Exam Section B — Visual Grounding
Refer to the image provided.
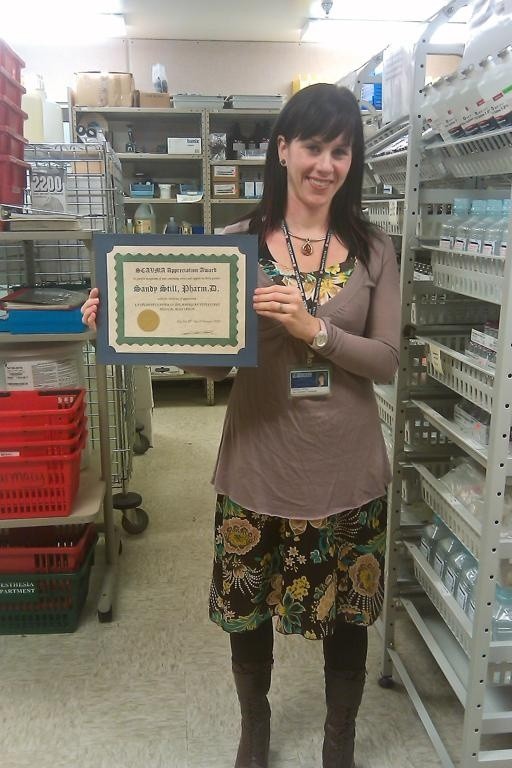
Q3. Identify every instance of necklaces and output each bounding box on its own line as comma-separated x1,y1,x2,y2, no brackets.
281,218,338,259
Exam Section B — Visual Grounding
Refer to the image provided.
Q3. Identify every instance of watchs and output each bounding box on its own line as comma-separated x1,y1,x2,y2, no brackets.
307,317,328,351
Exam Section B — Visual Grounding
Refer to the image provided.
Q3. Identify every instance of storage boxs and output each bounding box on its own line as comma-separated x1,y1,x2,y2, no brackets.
1,39,31,204
74,70,133,107
212,165,240,181
138,91,170,108
212,182,240,199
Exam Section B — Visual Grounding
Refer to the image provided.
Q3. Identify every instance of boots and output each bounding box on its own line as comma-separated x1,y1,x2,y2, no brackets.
322,666,365,768
233,665,271,767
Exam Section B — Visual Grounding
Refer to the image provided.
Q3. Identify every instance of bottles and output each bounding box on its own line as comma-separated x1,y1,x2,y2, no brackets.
420,516,512,684
420,45,512,150
438,194,512,254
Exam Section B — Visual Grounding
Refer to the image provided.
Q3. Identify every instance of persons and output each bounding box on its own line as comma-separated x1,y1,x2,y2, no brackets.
81,81,404,768
316,373,326,387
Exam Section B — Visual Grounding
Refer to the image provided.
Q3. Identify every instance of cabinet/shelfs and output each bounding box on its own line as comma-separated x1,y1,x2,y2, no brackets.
64,103,293,404
0,226,125,626
336,122,512,766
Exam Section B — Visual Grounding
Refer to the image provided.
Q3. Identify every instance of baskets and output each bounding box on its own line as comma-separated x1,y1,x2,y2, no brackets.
0,387,86,519
0,37,29,213
0,532,100,633
1,521,96,609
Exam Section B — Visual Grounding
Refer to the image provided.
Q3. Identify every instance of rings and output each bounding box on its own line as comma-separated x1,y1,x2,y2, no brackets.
278,301,285,314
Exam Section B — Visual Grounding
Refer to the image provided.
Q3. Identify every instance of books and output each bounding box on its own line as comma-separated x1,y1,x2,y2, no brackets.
0,285,90,313
